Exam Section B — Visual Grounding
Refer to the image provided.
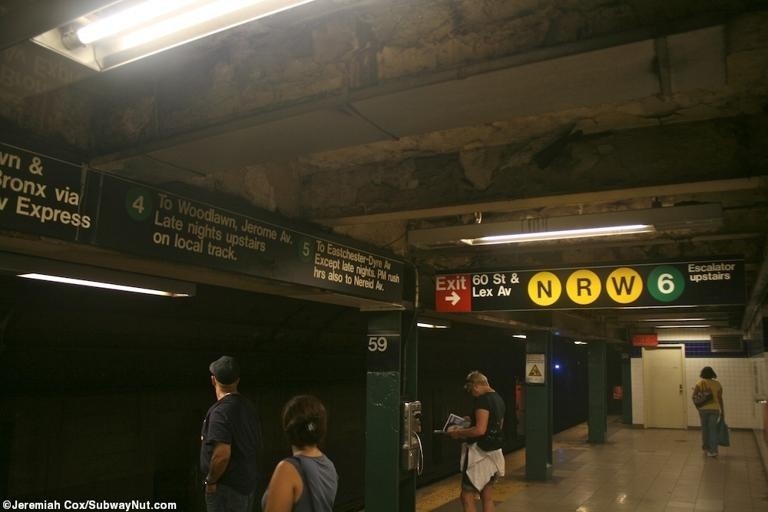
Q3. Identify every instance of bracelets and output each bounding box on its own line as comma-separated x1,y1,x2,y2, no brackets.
203,477,217,486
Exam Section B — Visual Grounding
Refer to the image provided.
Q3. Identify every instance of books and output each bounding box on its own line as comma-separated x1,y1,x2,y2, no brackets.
434,412,471,434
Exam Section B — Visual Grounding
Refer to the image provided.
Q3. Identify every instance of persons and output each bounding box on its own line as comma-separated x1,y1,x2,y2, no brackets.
447,370,506,511
445,423,461,432
692,366,724,458
260,396,340,511
198,355,260,511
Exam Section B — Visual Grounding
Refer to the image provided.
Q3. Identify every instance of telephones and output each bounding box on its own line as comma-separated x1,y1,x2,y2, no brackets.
401,400,422,471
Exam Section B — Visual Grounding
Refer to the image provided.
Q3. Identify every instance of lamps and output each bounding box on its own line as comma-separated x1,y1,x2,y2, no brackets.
407,204,724,249
0,251,197,297
30,0,320,72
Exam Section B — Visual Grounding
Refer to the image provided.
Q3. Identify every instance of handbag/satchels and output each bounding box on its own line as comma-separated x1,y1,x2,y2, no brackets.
693,380,713,406
717,417,729,446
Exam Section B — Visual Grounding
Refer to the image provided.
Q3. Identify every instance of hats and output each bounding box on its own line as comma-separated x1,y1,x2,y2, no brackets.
209,355,241,384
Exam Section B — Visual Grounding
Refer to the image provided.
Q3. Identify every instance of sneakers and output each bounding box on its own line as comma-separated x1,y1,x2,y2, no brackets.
707,451,718,457
